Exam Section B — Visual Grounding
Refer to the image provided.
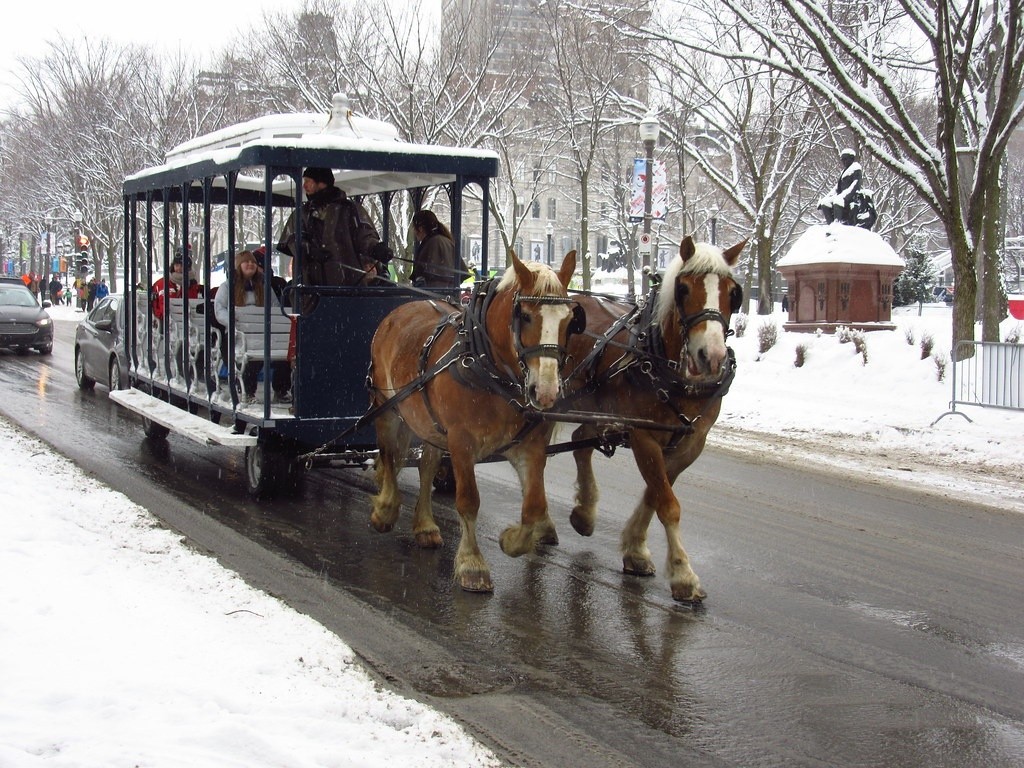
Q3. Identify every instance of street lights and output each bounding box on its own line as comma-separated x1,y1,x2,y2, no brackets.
638,109,662,293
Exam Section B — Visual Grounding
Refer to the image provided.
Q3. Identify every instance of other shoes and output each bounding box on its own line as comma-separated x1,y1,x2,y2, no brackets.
274,391,294,403
244,392,262,404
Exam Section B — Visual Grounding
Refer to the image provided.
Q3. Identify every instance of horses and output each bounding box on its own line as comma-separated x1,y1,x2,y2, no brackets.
370,235,753,604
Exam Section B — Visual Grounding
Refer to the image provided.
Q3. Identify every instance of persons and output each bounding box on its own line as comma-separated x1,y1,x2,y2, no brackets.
817,148,863,225
22,271,109,312
782,294,789,312
276,166,473,288
149,239,293,405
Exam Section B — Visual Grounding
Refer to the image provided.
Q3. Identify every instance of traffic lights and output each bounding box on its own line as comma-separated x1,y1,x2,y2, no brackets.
79,235,88,273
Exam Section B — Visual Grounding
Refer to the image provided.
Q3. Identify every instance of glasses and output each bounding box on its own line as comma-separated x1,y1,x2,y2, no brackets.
174,252,192,259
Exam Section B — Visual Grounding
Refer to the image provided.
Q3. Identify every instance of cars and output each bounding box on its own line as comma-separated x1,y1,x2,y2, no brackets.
73,291,129,401
0,277,54,355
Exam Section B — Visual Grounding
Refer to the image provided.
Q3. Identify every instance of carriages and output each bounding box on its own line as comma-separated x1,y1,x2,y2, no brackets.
107,92,753,605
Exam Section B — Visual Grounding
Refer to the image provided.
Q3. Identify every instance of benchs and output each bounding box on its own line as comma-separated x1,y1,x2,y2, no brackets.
135,292,292,411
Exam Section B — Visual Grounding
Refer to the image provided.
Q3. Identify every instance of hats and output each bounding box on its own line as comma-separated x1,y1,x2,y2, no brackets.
252,247,266,265
303,166,334,187
236,249,258,268
